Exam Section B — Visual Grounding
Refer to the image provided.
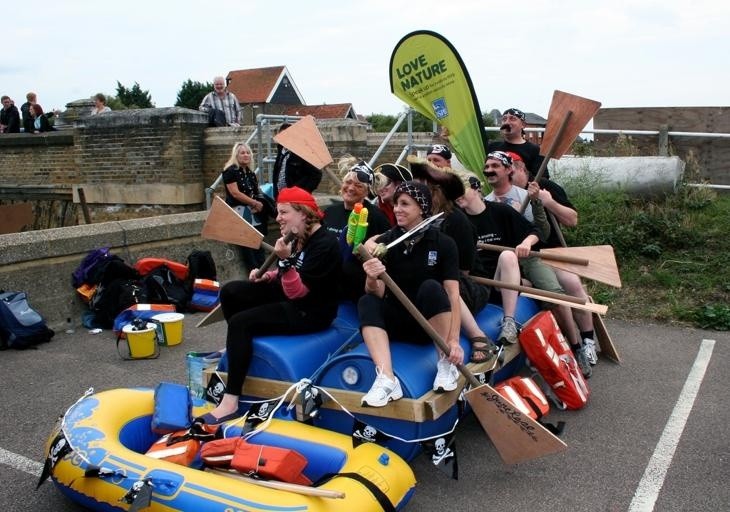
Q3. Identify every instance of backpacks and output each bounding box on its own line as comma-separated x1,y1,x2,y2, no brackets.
183,247,221,313
0,287,55,352
145,263,190,315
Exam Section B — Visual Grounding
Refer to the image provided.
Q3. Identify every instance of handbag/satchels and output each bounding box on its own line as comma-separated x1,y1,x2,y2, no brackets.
71,248,141,330
208,108,232,128
112,303,180,347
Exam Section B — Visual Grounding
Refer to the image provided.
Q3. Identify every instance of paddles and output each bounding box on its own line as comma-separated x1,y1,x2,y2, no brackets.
356,243,568,466
476,241,622,288
518,90,601,215
460,271,609,315
201,195,274,252
195,226,298,327
272,115,343,190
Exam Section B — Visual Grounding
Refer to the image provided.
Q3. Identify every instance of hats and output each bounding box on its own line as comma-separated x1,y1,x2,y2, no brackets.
371,162,415,184
277,183,315,203
406,154,466,202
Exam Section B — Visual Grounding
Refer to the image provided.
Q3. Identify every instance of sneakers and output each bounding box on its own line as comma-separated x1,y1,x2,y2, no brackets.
573,347,594,379
496,315,518,345
360,363,404,407
431,349,461,393
195,407,243,425
583,337,599,365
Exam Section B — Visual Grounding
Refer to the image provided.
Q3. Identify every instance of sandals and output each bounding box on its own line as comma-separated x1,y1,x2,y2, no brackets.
468,335,498,363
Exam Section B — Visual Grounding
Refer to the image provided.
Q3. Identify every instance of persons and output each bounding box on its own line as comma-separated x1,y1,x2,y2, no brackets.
323,144,542,408
21,93,37,132
195,186,343,425
224,124,322,273
90,93,111,116
483,108,598,378
28,103,52,134
199,75,242,127
0,95,20,133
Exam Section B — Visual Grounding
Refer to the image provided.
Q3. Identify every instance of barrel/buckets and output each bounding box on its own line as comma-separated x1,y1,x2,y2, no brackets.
152,312,185,346
120,321,159,358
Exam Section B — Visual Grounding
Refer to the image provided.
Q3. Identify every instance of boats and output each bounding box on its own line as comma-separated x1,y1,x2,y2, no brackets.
41,386,419,510
200,286,547,463
